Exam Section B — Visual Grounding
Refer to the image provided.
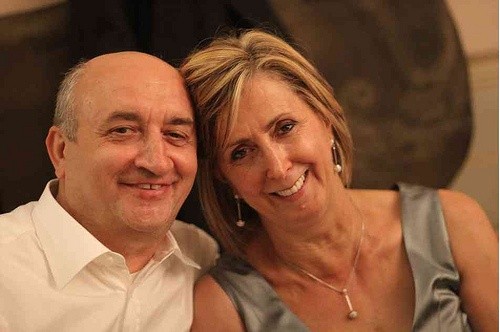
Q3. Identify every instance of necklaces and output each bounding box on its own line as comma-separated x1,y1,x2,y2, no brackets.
282,190,365,319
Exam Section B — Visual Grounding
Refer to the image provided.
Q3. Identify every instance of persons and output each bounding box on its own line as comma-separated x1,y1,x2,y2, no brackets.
0,51,220,332
178,22,500,332
